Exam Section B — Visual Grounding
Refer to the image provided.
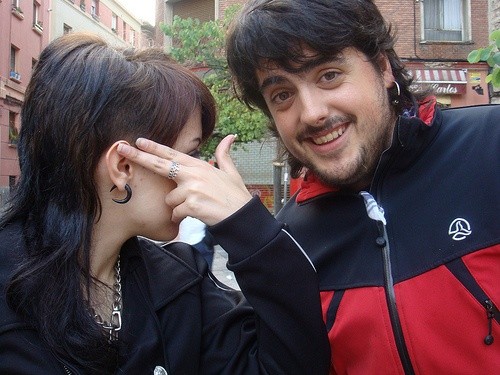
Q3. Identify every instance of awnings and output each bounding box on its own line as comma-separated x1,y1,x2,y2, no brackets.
404,68,467,95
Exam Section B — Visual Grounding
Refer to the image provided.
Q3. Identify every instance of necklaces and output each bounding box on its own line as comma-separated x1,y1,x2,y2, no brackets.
76,258,125,342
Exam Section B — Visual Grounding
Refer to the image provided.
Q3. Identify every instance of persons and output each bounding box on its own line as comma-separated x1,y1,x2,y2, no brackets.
0,34,334,375
226,0,500,375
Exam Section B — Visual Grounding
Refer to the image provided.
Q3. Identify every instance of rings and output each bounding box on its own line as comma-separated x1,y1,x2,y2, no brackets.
169,161,181,181
